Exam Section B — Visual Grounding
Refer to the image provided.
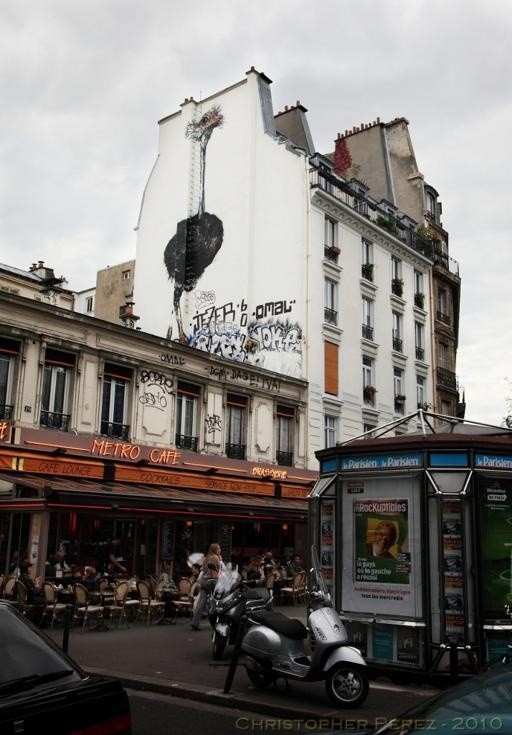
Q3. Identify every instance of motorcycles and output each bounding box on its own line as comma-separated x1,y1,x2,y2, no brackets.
240,587,370,710
203,563,277,660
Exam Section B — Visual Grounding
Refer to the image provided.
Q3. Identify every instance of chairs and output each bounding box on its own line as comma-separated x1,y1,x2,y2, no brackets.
0,557,309,635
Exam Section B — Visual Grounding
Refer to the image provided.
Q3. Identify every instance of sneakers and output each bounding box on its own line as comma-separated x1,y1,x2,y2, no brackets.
191,624,199,631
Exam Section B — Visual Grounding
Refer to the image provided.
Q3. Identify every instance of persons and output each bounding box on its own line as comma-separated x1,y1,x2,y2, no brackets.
192,541,224,634
368,522,399,560
1,550,309,625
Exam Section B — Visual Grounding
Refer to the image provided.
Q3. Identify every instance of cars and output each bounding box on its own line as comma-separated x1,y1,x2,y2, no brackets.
378,657,511,734
1,602,135,735
